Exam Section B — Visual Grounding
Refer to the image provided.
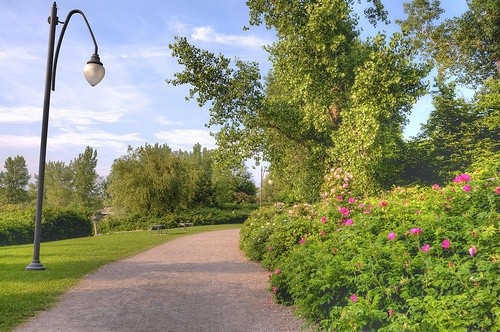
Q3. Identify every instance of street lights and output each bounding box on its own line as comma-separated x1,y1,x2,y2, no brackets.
24,2,106,275
259,164,270,207
